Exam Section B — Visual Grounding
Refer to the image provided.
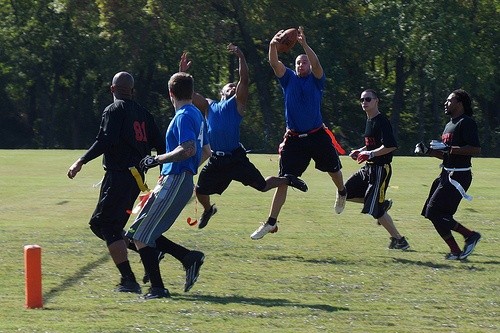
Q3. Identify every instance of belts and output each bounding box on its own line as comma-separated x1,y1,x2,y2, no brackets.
289,126,324,137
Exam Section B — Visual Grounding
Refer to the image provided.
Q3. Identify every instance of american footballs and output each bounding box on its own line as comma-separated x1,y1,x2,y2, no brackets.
278,28,299,53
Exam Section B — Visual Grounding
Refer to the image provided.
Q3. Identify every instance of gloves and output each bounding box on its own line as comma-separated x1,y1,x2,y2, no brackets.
414,143,430,154
430,140,453,154
357,151,373,164
140,155,161,174
349,149,362,160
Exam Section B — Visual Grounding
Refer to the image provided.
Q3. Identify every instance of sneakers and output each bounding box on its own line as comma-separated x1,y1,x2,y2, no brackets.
250,221,278,239
387,236,410,251
444,251,466,261
284,174,308,192
378,199,392,225
114,276,142,295
463,231,481,256
335,189,347,214
138,287,170,300
199,203,217,229
139,251,166,284
182,250,205,292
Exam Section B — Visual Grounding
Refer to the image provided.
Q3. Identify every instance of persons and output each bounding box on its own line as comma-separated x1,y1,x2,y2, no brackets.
341,89,410,251
67,72,166,292
422,89,482,261
177,43,309,229
248,27,346,241
124,72,205,299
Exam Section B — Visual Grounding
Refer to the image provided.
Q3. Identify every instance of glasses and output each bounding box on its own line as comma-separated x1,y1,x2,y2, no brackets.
359,97,376,102
